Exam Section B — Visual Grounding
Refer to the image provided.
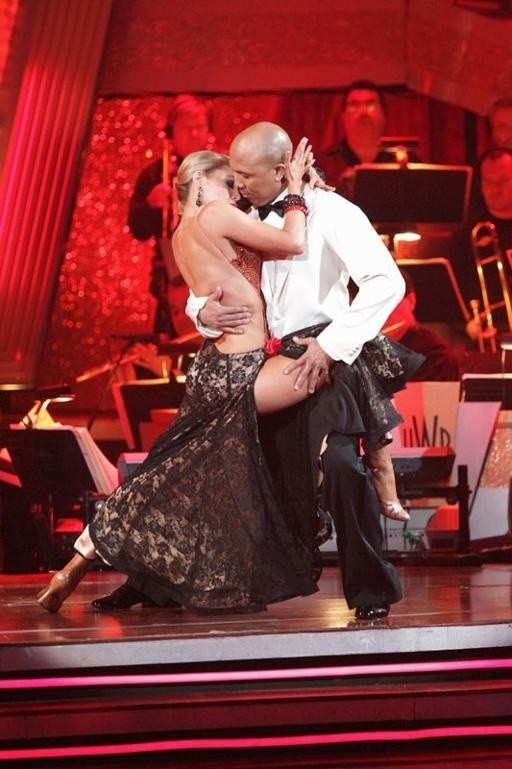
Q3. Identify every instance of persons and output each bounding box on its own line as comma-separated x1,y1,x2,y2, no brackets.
35,135,413,615
91,120,410,619
483,94,511,154
125,94,213,337
308,76,424,190
441,142,512,308
125,271,209,378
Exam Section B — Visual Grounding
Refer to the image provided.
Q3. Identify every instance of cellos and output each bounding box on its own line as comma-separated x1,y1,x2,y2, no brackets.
155,123,208,352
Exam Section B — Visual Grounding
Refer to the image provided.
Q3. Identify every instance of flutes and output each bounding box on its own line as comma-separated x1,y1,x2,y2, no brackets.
75,346,154,383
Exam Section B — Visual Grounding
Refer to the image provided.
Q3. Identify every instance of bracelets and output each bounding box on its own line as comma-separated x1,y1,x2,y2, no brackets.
282,194,309,217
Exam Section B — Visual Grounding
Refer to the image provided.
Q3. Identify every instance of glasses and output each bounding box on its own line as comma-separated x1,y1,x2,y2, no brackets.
343,97,379,113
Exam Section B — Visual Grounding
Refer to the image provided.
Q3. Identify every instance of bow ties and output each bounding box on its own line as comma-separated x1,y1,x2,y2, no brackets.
256,200,288,220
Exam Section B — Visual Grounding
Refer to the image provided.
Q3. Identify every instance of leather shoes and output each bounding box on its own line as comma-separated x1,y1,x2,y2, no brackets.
92,583,152,610
366,458,410,523
354,601,390,620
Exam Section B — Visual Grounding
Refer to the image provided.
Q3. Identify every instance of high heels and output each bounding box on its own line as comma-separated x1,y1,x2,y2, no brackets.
36,568,74,613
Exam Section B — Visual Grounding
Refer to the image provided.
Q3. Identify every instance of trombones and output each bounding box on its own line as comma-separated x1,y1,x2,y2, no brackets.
471,221,512,352
